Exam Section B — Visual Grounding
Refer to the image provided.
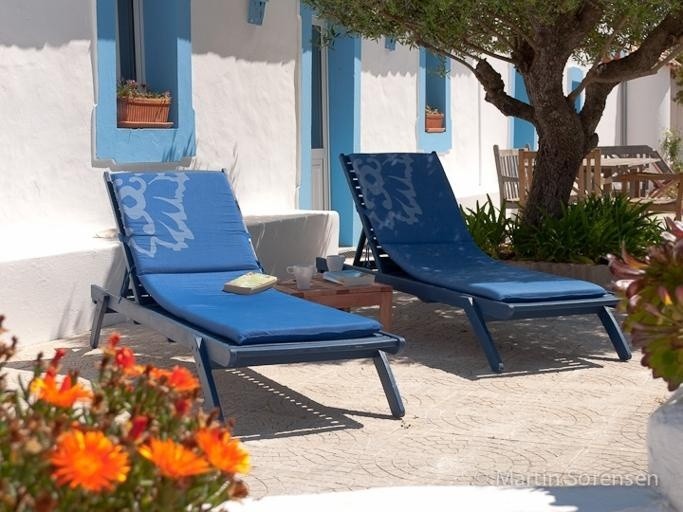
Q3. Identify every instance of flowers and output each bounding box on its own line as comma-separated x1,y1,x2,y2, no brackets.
1,314,253,511
116,77,170,99
425,104,441,114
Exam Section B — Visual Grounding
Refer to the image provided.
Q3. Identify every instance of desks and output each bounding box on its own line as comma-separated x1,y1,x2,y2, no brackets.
269,275,392,333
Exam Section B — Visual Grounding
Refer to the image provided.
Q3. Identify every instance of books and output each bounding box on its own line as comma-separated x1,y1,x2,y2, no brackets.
322,268,375,287
223,272,277,295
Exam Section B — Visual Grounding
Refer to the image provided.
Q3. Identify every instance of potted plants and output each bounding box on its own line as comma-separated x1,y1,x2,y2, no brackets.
607,216,683,512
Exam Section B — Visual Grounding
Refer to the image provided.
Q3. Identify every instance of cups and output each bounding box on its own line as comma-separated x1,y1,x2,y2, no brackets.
326,255,346,273
287,264,314,290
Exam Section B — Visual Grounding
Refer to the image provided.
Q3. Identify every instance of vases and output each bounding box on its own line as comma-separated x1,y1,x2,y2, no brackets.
425,113,446,133
117,96,175,128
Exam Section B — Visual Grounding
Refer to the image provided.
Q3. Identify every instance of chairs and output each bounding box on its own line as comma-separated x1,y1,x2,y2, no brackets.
340,150,634,372
87,168,405,433
492,143,681,248
